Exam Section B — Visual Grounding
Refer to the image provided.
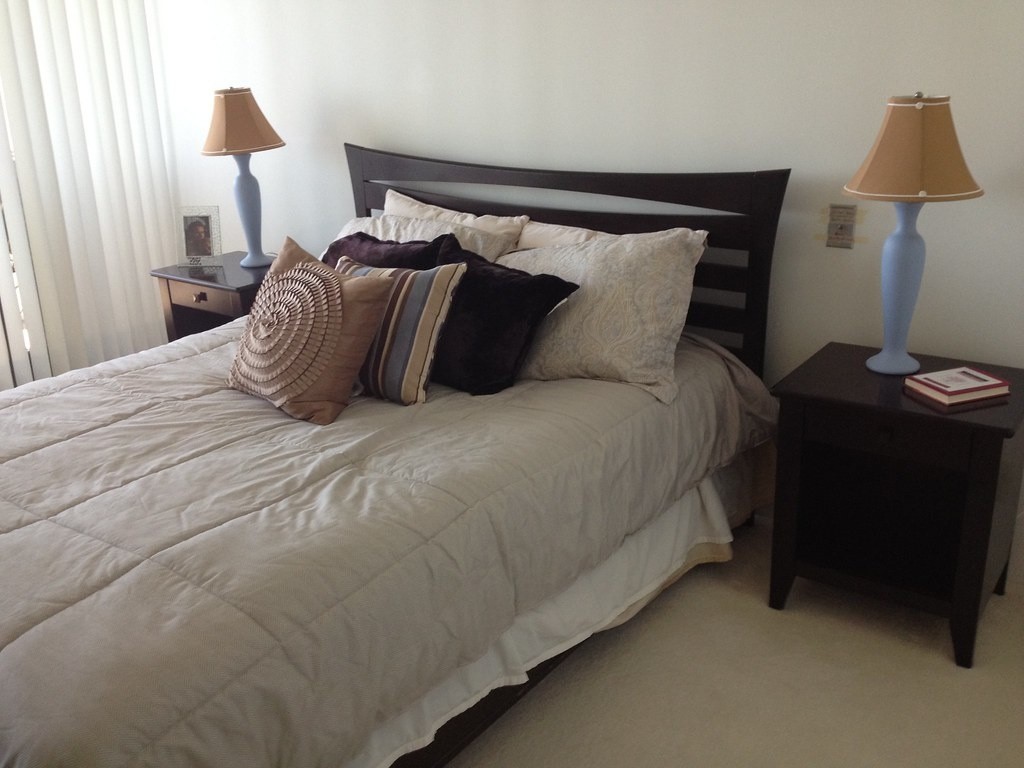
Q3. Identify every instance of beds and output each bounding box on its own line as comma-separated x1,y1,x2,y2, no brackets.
0,143,793,768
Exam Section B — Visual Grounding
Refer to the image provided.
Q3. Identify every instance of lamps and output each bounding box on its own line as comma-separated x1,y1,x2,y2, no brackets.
841,91,984,375
201,87,287,268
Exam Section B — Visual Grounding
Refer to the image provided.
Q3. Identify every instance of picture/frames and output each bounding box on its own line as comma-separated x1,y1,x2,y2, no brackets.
174,205,223,268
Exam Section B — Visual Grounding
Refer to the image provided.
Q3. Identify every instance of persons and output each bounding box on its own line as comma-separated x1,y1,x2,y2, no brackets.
185,218,211,256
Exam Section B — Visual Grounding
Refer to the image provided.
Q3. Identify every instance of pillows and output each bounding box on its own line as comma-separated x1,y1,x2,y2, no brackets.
225,235,396,425
495,227,710,406
435,233,580,397
318,189,530,263
334,255,468,407
321,230,448,271
518,220,613,250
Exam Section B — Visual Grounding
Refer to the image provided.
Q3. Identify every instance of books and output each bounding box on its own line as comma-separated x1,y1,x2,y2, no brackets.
902,366,1011,407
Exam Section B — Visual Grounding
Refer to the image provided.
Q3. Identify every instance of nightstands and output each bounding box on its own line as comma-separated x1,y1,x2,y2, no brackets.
769,341,1024,669
150,251,273,343
